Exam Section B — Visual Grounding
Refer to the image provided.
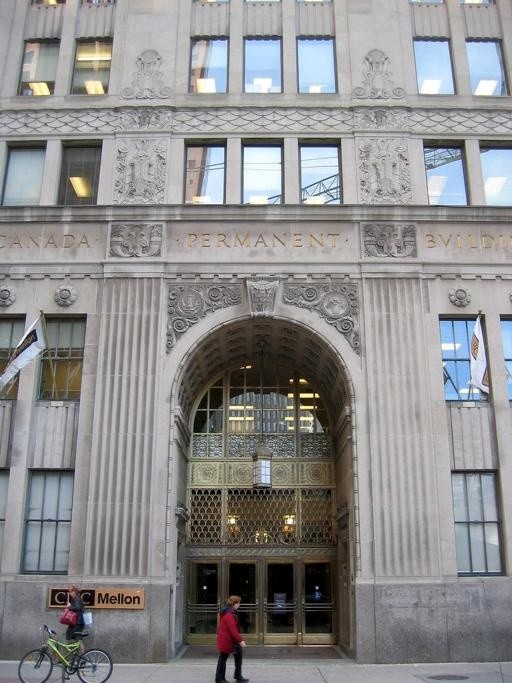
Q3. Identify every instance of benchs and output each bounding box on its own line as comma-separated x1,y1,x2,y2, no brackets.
0,314,46,390
470,316,490,394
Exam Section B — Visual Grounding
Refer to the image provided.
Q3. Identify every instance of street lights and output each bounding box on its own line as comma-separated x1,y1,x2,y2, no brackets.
83,612,93,626
58,608,79,626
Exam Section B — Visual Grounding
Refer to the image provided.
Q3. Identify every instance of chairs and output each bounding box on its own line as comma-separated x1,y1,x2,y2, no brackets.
216,679,229,683
234,674,248,682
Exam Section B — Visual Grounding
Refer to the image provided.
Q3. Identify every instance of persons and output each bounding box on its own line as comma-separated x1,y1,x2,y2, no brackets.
215,596,249,683
66,586,84,656
